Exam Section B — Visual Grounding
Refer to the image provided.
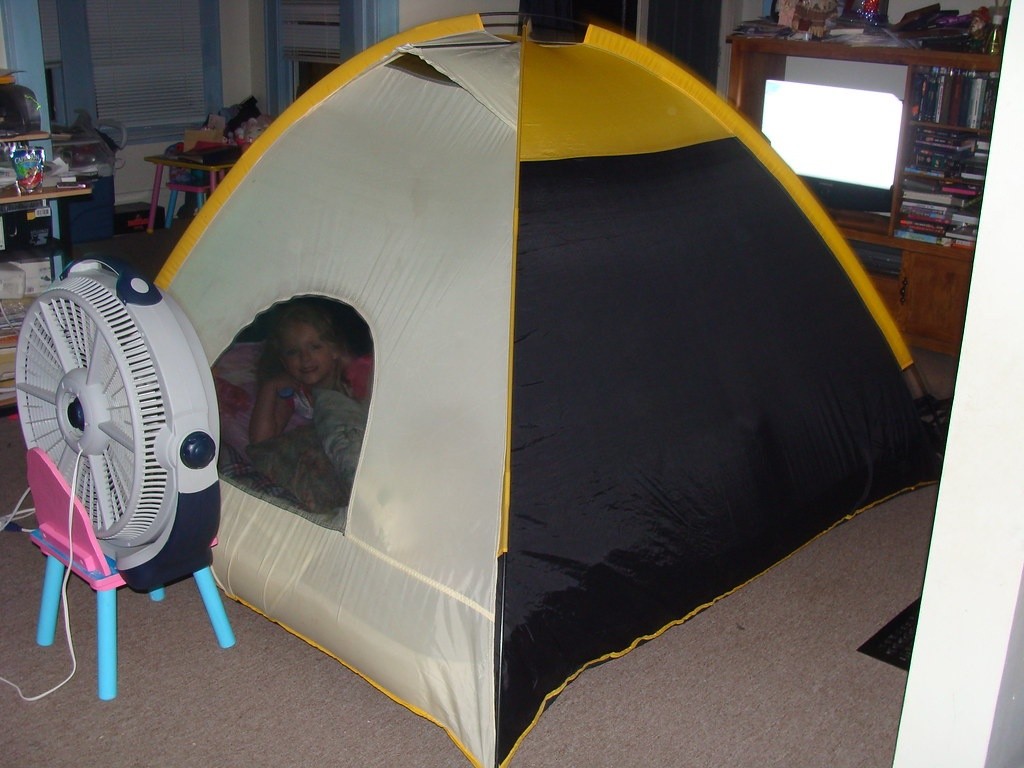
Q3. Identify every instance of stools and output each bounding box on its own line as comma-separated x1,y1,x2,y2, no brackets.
166,182,209,229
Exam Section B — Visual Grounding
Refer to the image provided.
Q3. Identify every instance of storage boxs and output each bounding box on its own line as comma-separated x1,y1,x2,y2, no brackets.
57,176,166,245
8,256,52,295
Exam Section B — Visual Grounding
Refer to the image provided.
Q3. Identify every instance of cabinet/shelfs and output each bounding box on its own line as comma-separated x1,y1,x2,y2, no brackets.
0,132,93,203
725,34,1003,359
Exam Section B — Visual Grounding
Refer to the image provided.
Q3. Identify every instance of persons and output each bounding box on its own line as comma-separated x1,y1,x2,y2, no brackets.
246,300,375,445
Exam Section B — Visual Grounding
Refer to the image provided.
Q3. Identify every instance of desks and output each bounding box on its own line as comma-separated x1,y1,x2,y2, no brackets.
144,155,237,233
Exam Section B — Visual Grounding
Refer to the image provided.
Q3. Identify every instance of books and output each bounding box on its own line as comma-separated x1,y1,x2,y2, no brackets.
822,10,903,48
0,295,48,404
894,64,999,249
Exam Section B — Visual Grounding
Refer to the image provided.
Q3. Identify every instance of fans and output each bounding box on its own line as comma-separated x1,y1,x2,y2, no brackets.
15,250,222,593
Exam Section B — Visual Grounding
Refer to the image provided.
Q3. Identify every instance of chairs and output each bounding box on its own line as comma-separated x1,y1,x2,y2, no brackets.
25,447,237,701
